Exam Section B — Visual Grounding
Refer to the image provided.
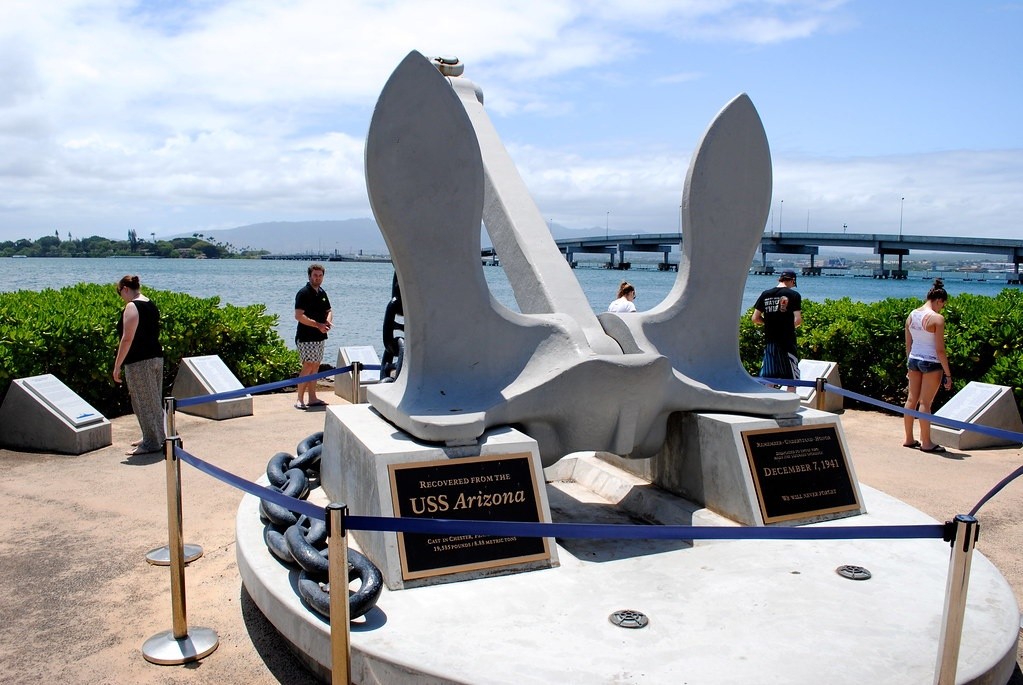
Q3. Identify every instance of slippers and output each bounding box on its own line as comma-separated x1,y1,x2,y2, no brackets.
295,402,309,409
903,440,921,448
920,445,946,453
306,401,329,406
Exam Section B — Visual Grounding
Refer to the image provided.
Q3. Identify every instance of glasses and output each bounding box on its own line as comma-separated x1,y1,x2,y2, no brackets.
633,293,636,299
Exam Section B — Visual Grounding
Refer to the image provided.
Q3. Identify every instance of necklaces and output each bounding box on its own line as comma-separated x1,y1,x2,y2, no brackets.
129,292,141,301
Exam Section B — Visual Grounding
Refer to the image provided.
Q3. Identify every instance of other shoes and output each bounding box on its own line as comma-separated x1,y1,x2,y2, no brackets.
126,437,161,456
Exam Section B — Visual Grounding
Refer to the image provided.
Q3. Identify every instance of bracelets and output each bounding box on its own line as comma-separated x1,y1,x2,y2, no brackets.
946,374,952,378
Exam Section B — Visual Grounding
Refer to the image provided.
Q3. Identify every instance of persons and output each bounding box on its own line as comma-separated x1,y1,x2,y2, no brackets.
605,280,637,314
293,262,332,413
111,274,169,457
902,279,954,453
750,266,804,396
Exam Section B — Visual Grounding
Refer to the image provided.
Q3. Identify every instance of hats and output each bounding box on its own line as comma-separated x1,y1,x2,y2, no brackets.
781,269,797,288
